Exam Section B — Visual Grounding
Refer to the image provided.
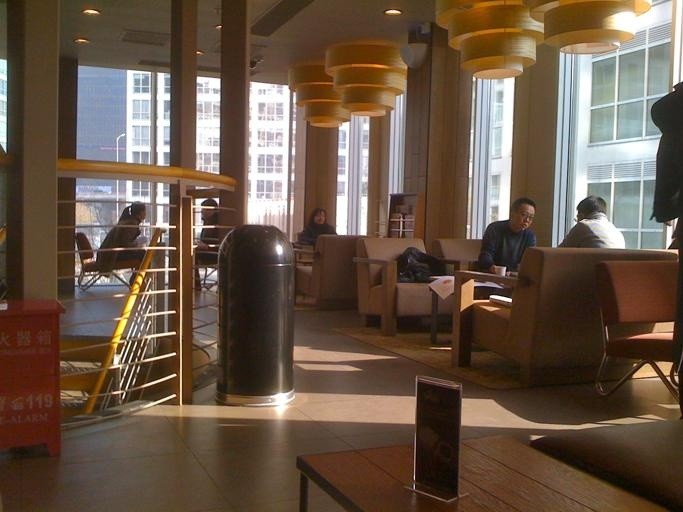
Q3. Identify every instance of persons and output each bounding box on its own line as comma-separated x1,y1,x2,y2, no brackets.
298,208,337,247
193,198,218,289
556,196,625,249
476,198,536,300
96,202,146,288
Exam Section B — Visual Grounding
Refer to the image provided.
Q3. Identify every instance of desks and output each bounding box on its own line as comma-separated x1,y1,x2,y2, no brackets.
293,436,672,511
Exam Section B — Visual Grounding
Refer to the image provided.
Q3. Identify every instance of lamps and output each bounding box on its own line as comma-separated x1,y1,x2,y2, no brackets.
287,66,351,128
435,0,544,80
527,0,651,54
326,44,407,118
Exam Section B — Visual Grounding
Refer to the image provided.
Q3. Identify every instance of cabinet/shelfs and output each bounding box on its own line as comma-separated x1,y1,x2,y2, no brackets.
386,192,425,240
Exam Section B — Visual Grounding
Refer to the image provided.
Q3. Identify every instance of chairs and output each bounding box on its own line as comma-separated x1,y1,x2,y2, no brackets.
593,259,683,412
352,237,460,336
432,238,483,275
294,232,369,308
199,264,219,294
74,230,139,291
450,247,679,385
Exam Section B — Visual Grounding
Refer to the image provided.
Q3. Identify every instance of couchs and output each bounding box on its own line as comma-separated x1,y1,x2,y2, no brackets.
528,419,681,512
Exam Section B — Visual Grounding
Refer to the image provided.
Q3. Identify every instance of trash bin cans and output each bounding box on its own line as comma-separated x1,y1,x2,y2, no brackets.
215,224,295,406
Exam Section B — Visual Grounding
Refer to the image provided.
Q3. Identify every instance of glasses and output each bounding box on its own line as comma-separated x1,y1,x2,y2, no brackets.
574,216,580,221
521,212,535,221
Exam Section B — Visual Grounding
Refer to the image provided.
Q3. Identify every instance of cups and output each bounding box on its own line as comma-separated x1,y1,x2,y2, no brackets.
493,265,507,277
